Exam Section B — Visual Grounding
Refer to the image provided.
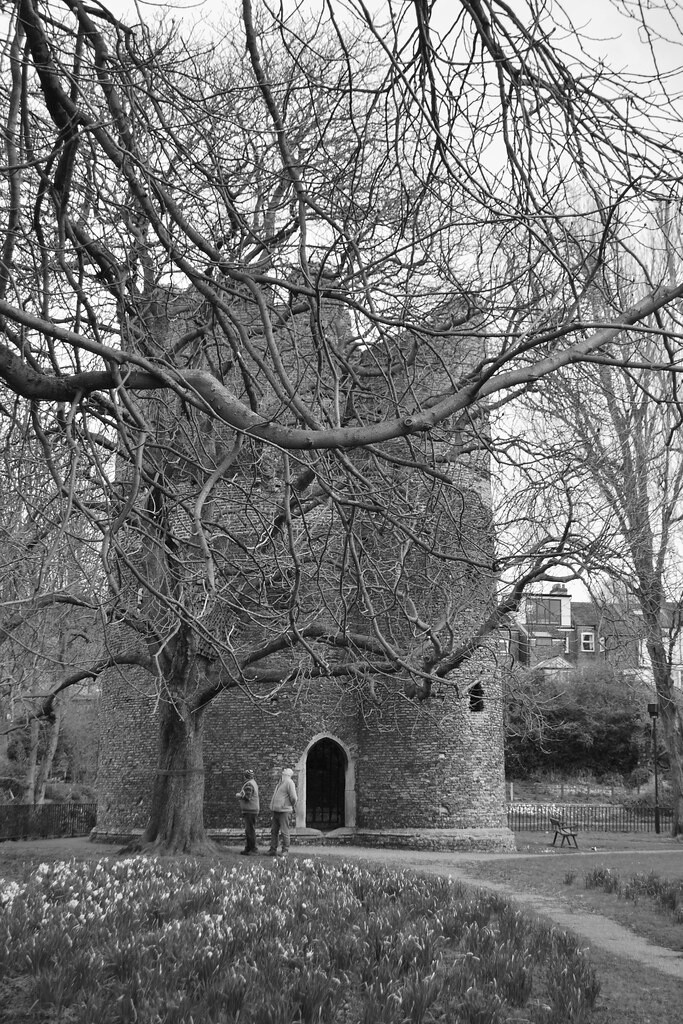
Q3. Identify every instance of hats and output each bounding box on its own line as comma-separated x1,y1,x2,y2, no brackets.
245,769,255,780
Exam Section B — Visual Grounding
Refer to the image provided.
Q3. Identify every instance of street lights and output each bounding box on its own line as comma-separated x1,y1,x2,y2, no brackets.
648,703,660,834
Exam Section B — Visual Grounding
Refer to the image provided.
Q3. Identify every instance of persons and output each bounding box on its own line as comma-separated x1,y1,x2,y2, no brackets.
264,768,297,857
235,770,260,856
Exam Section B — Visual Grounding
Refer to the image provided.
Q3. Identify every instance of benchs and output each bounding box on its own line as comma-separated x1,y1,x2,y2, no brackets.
549,817,579,849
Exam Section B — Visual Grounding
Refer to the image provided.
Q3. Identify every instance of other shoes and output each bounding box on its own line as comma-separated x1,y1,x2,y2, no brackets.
278,851,289,859
264,850,276,857
241,849,252,856
253,848,259,854
